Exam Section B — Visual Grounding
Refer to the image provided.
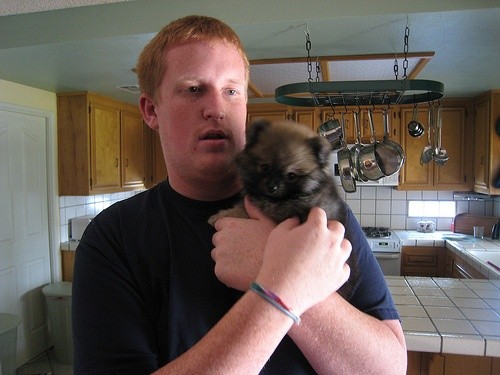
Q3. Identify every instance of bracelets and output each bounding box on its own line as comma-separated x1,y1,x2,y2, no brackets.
249,281,302,325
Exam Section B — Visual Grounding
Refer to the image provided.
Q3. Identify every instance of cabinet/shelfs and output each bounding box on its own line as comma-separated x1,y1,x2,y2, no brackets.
57,92,168,196
401,246,487,279
247,89,500,195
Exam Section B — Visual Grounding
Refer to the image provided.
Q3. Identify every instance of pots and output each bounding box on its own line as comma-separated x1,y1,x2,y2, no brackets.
316,108,405,193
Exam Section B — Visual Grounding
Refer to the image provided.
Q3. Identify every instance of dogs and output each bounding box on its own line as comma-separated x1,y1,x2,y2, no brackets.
208,117,338,227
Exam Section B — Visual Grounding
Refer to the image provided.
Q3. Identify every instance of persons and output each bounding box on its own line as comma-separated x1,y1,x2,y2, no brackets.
72,15,408,375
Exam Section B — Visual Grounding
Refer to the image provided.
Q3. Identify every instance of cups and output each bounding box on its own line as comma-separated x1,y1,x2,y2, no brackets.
473,226,484,239
416,221,435,233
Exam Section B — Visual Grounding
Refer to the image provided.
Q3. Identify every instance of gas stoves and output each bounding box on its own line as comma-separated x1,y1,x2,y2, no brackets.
361,225,403,252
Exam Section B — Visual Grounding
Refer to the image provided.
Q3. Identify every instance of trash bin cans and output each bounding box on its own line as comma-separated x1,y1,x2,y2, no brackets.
44,280,74,364
0,313,21,374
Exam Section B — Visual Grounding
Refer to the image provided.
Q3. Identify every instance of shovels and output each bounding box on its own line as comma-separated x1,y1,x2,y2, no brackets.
422,107,448,163
